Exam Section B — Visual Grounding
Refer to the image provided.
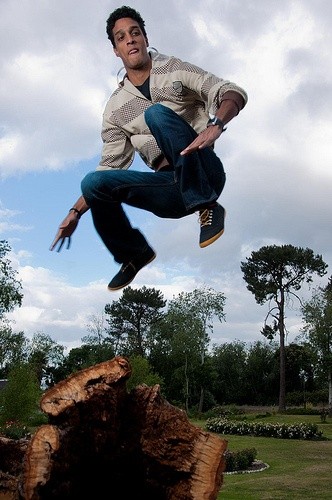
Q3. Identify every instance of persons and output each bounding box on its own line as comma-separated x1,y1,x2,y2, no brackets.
49,5,248,291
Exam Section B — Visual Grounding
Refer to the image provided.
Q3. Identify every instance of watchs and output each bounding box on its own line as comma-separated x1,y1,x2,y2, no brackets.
206,116,227,132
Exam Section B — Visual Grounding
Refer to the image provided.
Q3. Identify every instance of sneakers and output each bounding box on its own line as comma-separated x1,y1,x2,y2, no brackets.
108,247,156,290
199,202,226,248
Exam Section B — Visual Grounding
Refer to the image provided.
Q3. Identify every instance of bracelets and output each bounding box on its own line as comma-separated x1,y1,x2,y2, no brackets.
69,208,82,219
